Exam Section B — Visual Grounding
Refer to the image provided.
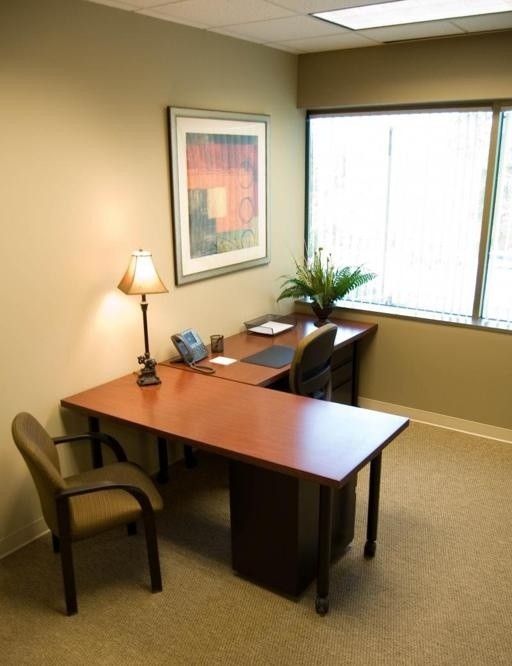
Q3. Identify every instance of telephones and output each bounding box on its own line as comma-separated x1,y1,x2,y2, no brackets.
170,328,208,365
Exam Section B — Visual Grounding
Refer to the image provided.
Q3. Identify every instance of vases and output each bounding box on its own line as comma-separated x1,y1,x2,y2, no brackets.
277,242,380,327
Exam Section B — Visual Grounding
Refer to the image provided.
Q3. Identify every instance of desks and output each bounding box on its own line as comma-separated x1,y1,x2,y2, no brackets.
61,312,413,616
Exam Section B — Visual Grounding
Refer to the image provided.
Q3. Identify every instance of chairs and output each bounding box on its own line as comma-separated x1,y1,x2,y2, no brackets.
10,410,166,617
284,321,338,403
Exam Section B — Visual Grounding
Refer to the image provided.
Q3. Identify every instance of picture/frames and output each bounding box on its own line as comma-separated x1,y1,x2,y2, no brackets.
167,102,274,289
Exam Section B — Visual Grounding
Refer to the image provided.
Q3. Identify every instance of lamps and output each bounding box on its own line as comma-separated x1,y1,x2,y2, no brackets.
117,248,174,388
